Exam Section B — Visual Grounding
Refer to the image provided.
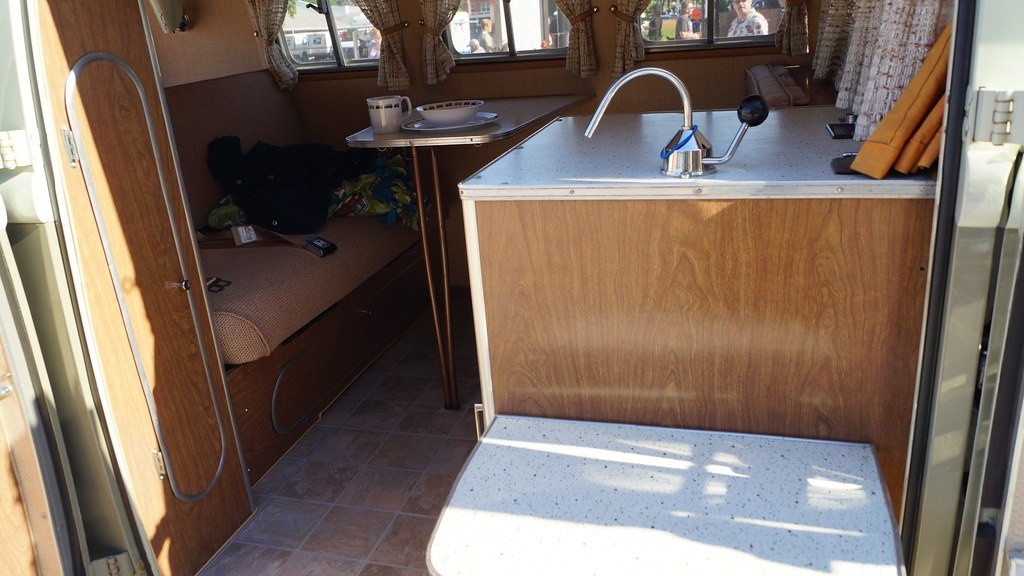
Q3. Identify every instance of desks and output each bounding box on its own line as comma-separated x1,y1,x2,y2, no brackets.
427,414,906,575
345,90,597,411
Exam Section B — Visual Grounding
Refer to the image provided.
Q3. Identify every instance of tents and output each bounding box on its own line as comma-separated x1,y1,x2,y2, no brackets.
281,1,374,35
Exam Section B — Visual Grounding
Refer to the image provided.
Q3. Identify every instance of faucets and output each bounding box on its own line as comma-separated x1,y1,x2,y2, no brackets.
582,67,770,179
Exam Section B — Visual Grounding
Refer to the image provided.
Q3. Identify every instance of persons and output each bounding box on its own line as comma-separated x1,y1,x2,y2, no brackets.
727,0,768,37
549,5,571,48
675,0,702,40
479,19,509,53
465,39,487,53
643,8,661,41
353,28,382,59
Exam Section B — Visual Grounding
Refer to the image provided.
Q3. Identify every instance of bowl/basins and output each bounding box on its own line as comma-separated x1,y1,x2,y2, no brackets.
414,100,484,128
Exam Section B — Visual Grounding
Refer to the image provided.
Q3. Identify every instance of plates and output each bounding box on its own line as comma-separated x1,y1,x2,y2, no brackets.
401,113,503,134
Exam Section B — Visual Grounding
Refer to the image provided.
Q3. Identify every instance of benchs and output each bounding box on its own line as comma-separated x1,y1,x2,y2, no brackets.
163,69,446,488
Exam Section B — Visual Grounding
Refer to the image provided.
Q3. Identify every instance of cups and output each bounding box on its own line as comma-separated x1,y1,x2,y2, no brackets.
366,95,412,135
